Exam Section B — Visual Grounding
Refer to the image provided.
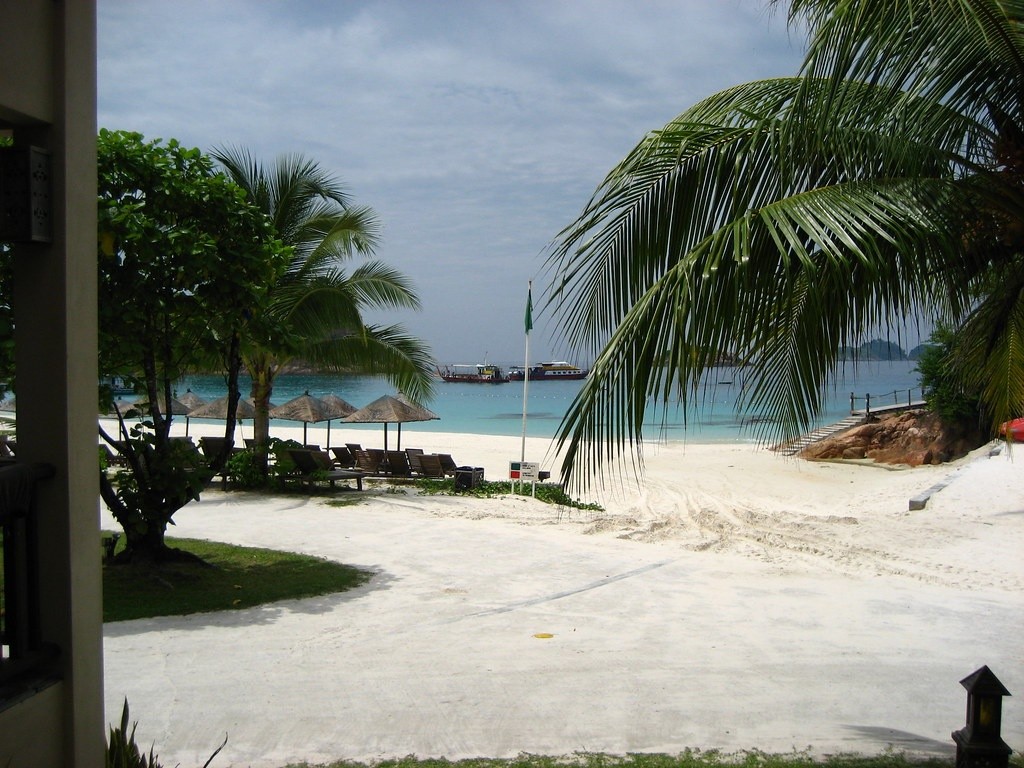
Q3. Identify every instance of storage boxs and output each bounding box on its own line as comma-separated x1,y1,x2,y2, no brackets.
453,467,485,489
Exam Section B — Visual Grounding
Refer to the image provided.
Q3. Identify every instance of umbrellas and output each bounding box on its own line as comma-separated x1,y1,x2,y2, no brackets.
117,391,194,417
184,395,277,448
174,388,208,438
268,390,359,453
98,397,134,442
0,397,16,413
340,394,441,463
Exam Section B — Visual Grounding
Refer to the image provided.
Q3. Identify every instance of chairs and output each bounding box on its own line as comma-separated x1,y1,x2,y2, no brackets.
99,437,457,491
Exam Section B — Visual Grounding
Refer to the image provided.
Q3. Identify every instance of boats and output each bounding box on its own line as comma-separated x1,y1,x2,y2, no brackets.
435,350,510,383
507,361,589,384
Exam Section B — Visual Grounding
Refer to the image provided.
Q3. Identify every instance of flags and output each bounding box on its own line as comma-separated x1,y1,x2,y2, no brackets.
523,295,534,335
